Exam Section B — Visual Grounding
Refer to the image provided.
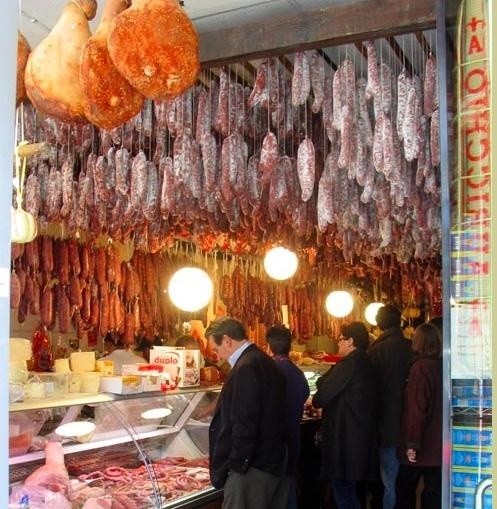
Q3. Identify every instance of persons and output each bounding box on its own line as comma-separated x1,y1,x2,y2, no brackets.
429,316,442,360
208,317,290,508
402,322,442,509
369,305,416,508
98,333,147,374
265,326,310,508
178,336,205,370
312,322,383,509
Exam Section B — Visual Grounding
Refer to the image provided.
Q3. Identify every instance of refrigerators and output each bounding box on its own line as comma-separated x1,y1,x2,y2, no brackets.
10,384,226,508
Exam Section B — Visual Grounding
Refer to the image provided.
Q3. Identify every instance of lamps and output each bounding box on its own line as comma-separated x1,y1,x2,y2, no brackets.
167,236,386,326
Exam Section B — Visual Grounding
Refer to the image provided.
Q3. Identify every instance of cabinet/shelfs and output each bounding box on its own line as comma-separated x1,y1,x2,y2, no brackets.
9,368,329,509
